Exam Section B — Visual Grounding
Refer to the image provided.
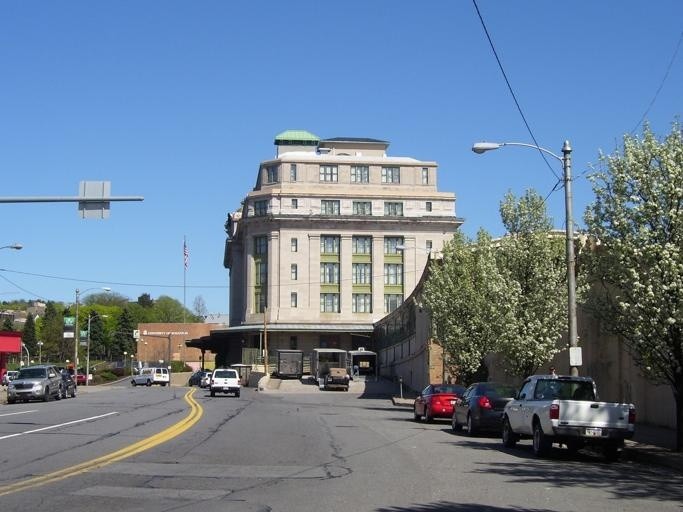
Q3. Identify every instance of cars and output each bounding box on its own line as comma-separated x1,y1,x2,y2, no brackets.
189,371,212,388
3,365,85,404
452,382,520,434
210,369,240,397
414,384,464,421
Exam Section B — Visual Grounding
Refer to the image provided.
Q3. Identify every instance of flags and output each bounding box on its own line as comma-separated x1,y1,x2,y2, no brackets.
184,239,189,269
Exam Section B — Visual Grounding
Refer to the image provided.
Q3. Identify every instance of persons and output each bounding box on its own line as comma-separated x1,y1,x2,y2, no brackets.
572,382,584,399
368,363,374,376
354,363,359,374
547,365,556,375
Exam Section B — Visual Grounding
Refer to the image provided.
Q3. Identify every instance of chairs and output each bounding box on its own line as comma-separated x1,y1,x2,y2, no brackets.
573,386,585,400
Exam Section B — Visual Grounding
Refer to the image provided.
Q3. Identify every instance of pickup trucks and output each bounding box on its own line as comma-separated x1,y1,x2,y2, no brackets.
503,374,635,455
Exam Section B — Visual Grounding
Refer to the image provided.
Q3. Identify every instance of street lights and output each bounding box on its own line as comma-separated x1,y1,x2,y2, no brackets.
86,315,107,385
473,141,580,375
74,288,109,386
38,342,43,363
131,355,134,367
138,339,147,367
124,352,127,368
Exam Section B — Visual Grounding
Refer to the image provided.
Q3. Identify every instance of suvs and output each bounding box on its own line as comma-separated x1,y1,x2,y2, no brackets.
131,368,169,386
